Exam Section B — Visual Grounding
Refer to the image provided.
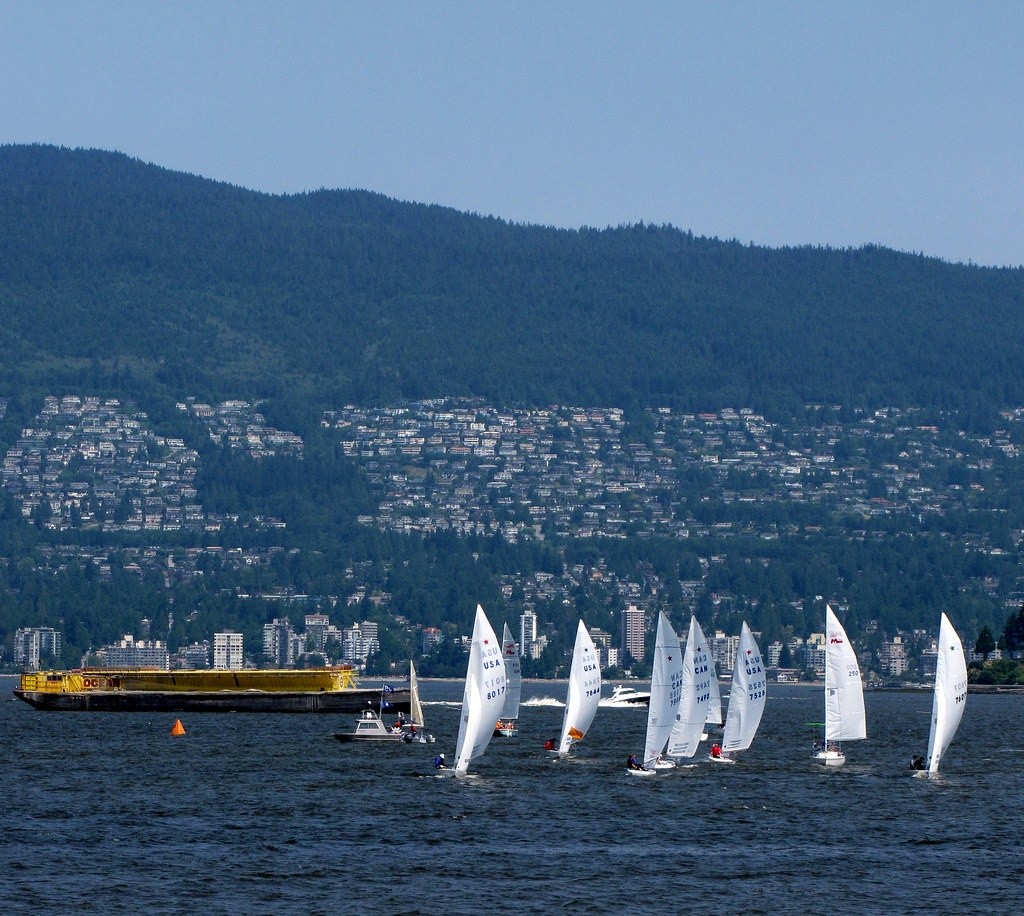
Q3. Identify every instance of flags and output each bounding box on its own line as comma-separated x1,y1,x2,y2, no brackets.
382,683,394,708
569,727,583,740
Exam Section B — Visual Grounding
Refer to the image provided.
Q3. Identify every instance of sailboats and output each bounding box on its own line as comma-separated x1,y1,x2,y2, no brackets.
398,601,970,782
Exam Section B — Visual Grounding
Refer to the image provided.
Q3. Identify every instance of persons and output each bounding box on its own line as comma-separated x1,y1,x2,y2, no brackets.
910,754,926,771
627,753,648,771
495,719,514,729
545,737,558,751
433,753,448,769
813,737,824,751
711,743,721,758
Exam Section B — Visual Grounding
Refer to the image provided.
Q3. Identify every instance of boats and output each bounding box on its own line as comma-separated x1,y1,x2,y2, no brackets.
14,666,410,713
332,710,406,742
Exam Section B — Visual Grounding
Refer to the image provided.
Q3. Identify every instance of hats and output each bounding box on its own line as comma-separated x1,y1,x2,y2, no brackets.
440,753,445,759
498,719,501,721
632,752,638,755
549,736,555,740
